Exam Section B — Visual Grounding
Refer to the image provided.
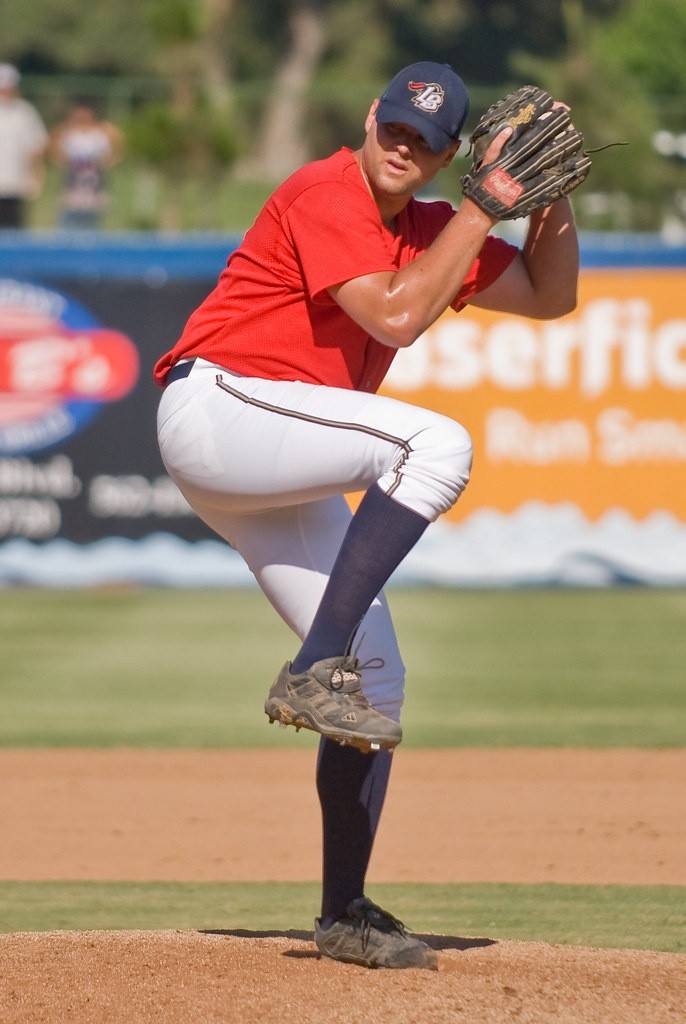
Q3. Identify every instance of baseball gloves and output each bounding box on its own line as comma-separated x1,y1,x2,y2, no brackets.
466,83,595,217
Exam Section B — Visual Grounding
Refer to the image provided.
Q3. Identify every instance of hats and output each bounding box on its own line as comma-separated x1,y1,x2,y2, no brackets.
375,61,469,153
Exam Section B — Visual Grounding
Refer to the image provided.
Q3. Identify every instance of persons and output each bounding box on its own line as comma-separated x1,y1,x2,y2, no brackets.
1,62,125,228
153,62,579,969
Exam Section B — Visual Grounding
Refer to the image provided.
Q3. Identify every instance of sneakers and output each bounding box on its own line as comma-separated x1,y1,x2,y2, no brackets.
314,896,438,969
263,615,403,753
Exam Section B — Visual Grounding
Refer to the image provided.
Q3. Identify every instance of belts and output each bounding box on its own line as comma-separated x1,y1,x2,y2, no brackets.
165,361,195,385
